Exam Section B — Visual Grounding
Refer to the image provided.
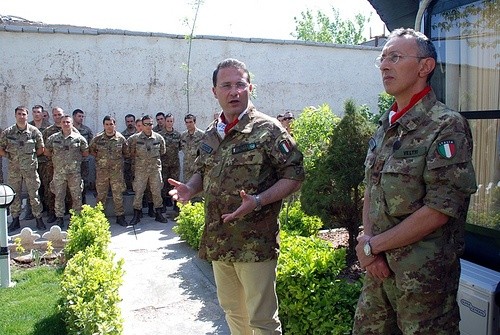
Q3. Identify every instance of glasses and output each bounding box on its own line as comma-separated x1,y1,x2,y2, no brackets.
375,52,427,68
215,82,250,91
143,123,153,126
284,117,293,121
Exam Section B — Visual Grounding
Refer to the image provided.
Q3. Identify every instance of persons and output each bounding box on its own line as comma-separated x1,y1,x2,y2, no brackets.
0,103,48,232
92,112,126,225
87,115,129,227
352,24,477,335
154,113,184,215
177,112,208,214
18,104,55,225
32,110,56,218
166,58,303,335
150,111,174,214
44,113,90,231
64,108,94,223
118,113,147,211
40,105,82,222
0,124,12,217
127,117,162,218
127,115,169,225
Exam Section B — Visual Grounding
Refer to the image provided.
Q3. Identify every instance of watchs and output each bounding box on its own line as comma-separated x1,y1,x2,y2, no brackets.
251,192,262,212
363,237,379,259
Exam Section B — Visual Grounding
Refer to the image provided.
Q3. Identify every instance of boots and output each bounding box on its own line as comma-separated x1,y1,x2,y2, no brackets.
166,197,172,207
173,200,179,210
36,217,46,230
130,209,141,225
7,217,20,233
116,215,128,226
25,214,34,220
142,201,148,208
155,208,168,223
47,215,56,222
160,204,168,212
138,209,143,219
56,218,65,229
148,202,155,217
